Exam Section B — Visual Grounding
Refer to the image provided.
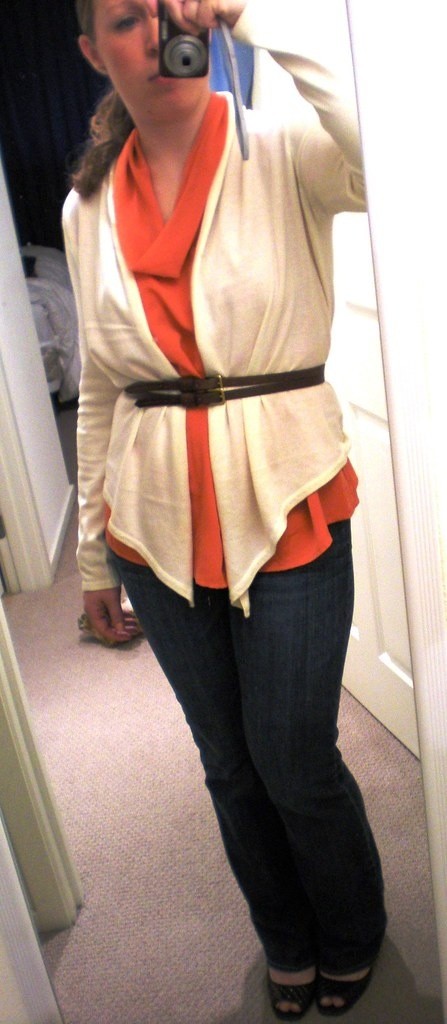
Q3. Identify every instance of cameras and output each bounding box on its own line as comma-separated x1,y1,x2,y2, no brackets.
157,0,210,79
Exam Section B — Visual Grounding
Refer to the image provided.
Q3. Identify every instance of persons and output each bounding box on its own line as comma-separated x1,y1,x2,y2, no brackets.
60,0,393,1024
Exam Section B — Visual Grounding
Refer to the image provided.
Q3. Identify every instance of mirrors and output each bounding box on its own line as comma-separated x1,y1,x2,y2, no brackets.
0,0,447,1024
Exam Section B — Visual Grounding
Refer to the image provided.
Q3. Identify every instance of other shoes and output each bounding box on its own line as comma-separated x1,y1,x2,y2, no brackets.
267,966,316,1020
314,959,372,1016
